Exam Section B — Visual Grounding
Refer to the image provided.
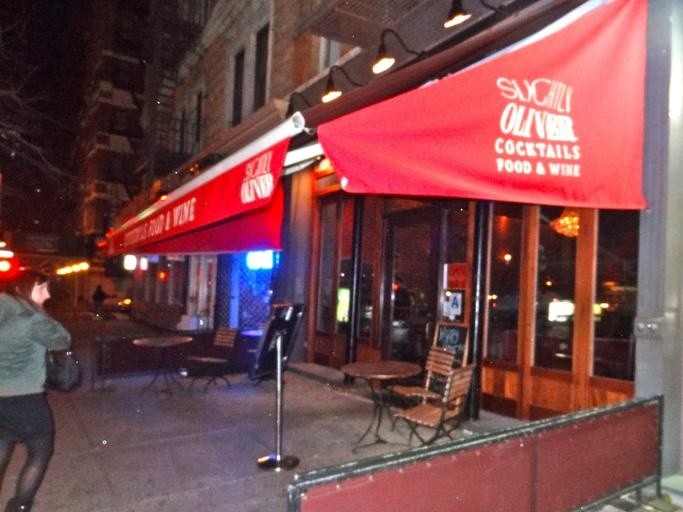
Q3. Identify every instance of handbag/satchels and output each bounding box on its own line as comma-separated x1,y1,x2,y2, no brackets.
44,350,82,392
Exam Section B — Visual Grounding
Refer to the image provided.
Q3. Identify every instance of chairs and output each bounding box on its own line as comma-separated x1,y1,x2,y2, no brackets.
183,325,240,393
390,363,480,449
385,345,457,410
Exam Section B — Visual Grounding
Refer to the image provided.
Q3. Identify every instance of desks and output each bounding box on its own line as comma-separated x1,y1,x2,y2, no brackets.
338,359,423,454
130,335,194,397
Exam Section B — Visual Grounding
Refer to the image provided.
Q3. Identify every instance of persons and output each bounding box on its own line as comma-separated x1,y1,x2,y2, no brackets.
0,270,71,512
541,285,563,315
391,275,421,360
91,284,106,317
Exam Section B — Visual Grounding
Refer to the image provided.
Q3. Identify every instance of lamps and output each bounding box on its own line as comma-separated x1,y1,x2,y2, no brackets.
284,91,313,120
547,207,580,240
442,0,509,29
369,27,432,75
318,62,365,104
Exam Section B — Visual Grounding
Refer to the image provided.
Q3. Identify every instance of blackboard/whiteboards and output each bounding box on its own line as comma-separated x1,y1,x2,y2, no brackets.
429,320,470,384
